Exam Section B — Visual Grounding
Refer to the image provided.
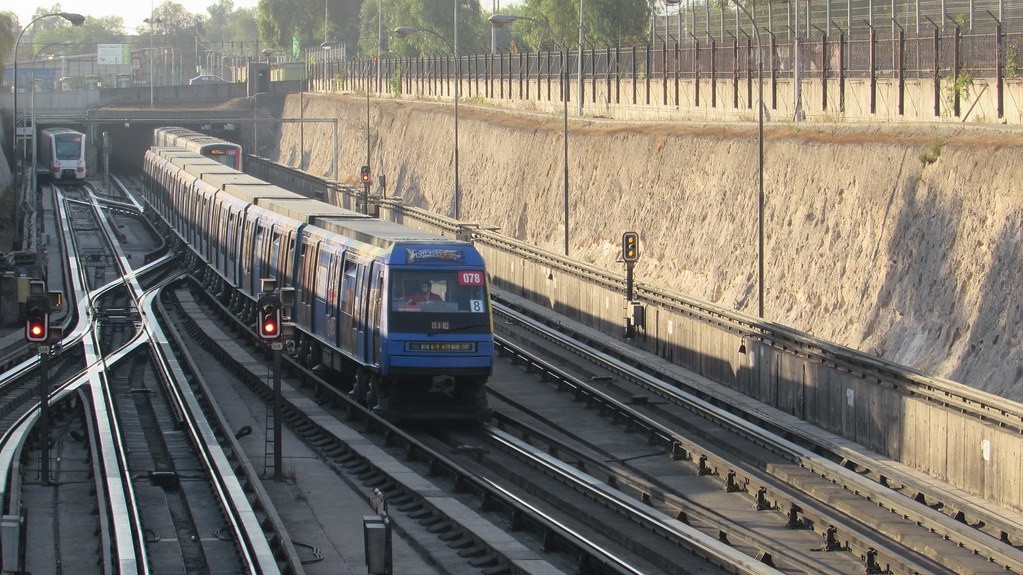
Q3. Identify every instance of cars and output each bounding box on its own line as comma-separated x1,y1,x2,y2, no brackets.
189,74,231,84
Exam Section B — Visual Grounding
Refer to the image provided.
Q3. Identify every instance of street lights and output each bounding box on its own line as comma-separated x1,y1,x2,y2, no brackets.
664,1,764,318
143,18,161,108
263,49,303,170
488,14,569,256
321,41,371,194
31,40,73,209
394,27,458,221
14,13,86,241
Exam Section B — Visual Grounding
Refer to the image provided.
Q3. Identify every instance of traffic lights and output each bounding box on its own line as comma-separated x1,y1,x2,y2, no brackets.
623,232,639,262
27,297,47,342
361,166,370,184
257,293,280,338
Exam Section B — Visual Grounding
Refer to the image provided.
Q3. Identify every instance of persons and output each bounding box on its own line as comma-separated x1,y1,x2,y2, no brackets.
410,281,442,305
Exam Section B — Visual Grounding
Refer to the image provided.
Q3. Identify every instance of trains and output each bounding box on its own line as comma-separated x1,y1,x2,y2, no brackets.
139,146,497,420
153,126,243,170
41,126,87,185
